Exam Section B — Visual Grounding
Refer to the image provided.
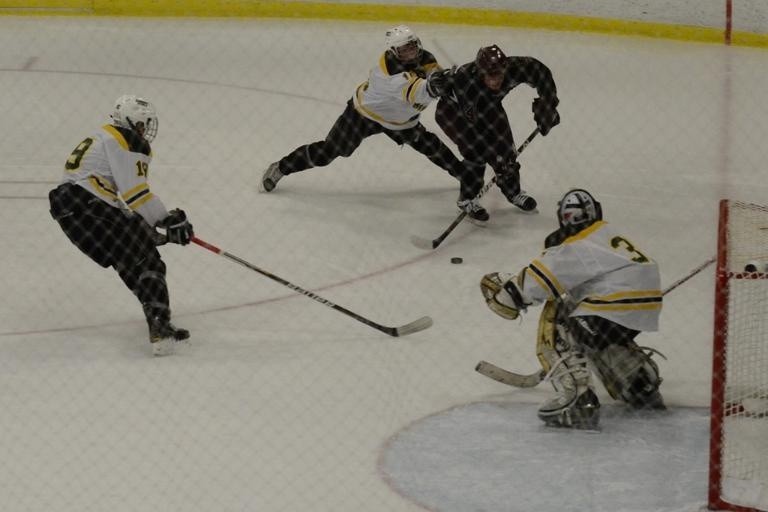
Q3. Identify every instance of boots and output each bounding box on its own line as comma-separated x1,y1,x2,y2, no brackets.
610,348,673,414
144,315,190,344
262,155,287,192
537,371,605,433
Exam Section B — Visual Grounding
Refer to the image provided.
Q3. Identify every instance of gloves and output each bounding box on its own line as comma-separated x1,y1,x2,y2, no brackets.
530,97,560,135
152,207,193,246
425,68,457,97
141,221,168,247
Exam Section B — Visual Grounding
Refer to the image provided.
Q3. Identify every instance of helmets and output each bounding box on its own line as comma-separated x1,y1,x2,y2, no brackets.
111,95,158,144
384,24,425,64
476,44,511,77
558,187,601,227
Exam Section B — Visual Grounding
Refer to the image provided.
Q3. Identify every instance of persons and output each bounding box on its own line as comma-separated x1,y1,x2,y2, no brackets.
263,25,463,192
479,187,664,429
48,94,191,347
434,44,560,221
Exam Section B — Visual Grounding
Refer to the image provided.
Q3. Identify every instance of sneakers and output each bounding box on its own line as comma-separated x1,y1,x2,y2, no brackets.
456,196,490,221
508,190,538,210
